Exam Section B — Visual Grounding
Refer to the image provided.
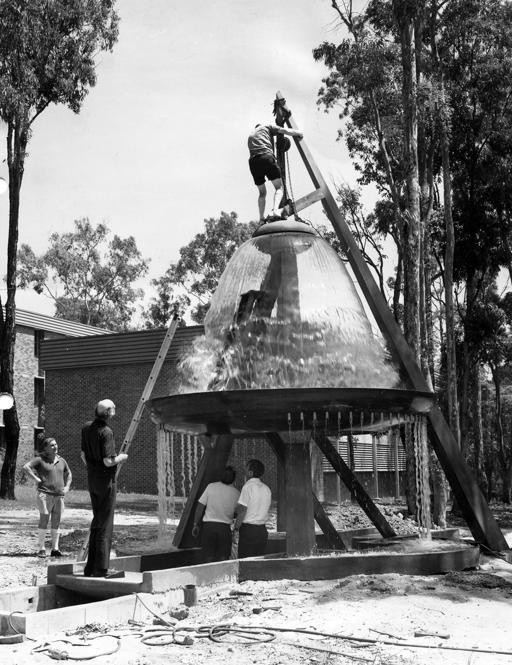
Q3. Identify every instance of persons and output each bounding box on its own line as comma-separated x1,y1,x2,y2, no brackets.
233,457,272,559
191,465,241,563
80,398,129,576
23,433,73,559
247,122,304,225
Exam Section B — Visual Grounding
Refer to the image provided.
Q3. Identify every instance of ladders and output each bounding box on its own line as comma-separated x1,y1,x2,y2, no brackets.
208,291,264,391
77,311,182,562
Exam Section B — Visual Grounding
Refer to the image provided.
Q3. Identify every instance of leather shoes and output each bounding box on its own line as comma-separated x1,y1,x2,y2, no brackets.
82,567,115,577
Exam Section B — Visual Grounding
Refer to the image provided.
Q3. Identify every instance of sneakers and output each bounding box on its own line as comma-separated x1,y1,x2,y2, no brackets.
51,550,67,557
36,550,47,557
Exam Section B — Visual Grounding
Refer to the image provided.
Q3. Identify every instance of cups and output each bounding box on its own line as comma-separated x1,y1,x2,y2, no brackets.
182,584,198,607
